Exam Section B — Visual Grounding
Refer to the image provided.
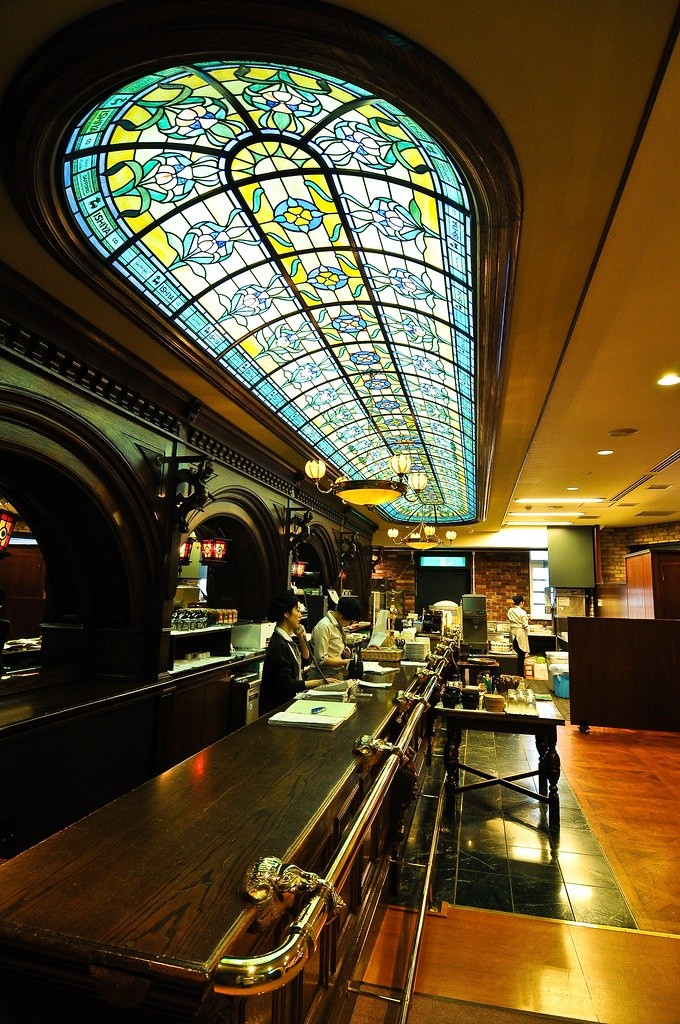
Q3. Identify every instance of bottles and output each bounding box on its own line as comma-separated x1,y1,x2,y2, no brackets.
171,610,208,631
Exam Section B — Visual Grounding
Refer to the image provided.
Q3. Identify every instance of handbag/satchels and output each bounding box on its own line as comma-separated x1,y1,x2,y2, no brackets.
348,652,363,679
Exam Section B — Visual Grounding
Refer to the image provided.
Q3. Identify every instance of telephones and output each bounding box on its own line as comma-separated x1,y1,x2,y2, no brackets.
293,623,305,637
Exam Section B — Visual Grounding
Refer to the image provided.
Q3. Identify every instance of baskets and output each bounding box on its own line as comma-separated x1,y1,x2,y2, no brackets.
361,646,404,661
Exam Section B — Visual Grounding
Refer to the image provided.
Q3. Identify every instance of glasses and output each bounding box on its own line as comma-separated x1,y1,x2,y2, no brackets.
349,620,354,627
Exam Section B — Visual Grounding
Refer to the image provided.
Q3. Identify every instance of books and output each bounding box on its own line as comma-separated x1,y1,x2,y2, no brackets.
268,679,359,731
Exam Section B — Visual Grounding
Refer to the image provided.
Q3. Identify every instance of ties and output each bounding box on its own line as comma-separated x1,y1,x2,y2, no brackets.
326,613,346,647
290,641,300,663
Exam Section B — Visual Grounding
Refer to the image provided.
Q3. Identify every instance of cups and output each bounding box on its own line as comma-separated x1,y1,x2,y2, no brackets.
507,689,536,713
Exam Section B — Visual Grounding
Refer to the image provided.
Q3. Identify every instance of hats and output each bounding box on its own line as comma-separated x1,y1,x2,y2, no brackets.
268,591,299,623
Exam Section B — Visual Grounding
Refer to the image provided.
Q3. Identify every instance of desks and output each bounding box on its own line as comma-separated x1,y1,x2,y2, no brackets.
434,679,565,832
457,661,499,685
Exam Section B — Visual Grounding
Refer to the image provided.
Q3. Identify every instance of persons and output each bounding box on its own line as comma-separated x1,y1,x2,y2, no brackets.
308,598,364,682
258,591,338,718
507,595,531,677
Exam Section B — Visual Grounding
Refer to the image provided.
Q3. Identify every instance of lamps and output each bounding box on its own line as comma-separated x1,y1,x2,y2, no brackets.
197,521,233,566
291,561,308,577
178,537,196,578
305,369,428,511
388,496,457,551
0,497,23,560
